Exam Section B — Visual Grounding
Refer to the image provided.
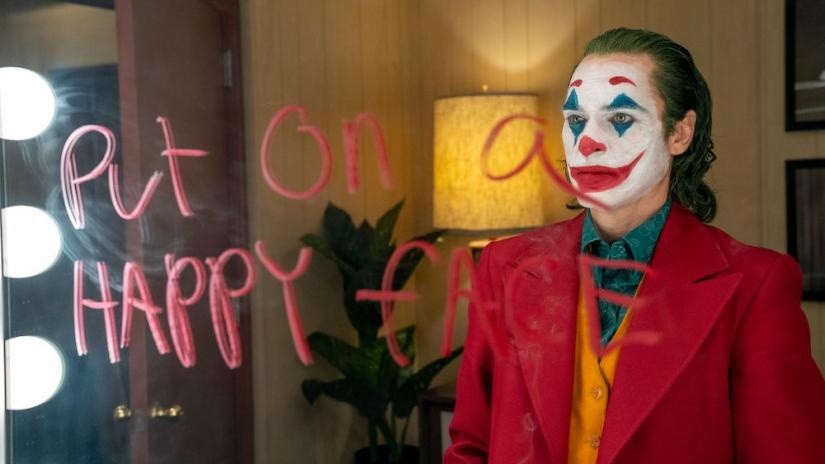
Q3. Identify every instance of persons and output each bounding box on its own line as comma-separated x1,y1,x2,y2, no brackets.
443,25,825,464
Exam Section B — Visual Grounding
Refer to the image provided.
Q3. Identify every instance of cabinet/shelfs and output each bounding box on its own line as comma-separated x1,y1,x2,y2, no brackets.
418,379,459,464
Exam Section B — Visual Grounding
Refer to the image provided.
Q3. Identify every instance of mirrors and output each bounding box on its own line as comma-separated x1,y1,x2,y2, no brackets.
0,0,825,464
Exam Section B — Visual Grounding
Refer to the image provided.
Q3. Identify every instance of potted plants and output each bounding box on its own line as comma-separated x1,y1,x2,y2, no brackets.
299,194,464,464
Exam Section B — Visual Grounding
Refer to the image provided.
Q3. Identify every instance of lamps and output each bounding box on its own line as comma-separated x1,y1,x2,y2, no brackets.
431,95,545,262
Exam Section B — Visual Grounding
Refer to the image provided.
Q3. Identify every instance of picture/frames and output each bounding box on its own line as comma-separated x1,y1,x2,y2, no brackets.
784,158,825,303
785,0,825,132
